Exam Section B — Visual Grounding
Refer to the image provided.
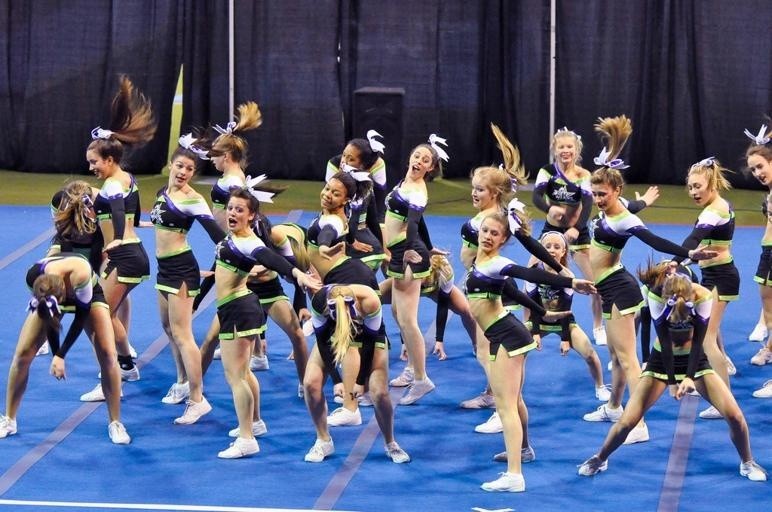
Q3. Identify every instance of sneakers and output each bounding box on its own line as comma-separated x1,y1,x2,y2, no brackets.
249,355,269,370
327,395,373,426
700,405,724,418
0,414,17,439
750,324,768,342
384,441,410,463
494,446,535,462
751,348,772,365
161,378,213,425
584,385,649,445
592,325,607,345
725,355,737,375
481,471,525,492
98,365,140,382
109,420,130,444
390,367,434,406
461,391,497,409
305,436,335,463
81,383,123,401
579,454,608,475
229,419,267,437
218,438,259,458
753,380,772,397
740,461,767,481
475,411,504,433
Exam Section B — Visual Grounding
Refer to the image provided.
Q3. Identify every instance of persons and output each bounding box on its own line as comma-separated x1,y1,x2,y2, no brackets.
460,115,772,492
150,103,476,464
0,74,154,444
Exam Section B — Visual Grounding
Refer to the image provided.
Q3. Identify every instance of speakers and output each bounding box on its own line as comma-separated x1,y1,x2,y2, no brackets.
354,85,406,192
